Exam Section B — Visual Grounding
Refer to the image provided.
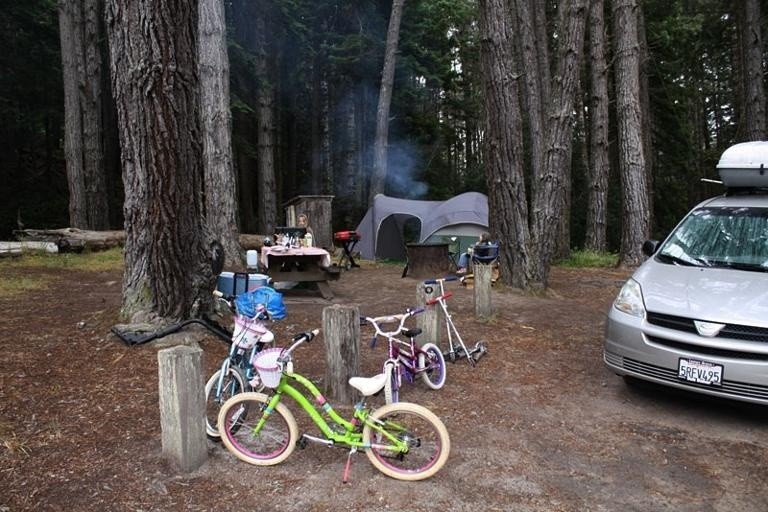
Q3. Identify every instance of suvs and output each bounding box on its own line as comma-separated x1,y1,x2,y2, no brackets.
603,142,767,403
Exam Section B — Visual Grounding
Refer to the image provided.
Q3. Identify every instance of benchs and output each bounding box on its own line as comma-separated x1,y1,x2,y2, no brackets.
320,265,340,281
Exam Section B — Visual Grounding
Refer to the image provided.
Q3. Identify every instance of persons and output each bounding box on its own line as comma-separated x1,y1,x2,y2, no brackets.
296,212,313,248
471,231,489,258
456,233,492,275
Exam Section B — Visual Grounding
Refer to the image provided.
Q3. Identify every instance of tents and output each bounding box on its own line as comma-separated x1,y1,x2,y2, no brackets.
345,191,489,264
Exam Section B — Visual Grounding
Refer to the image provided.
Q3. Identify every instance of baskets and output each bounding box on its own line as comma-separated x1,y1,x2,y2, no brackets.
232,317,265,350
253,347,287,388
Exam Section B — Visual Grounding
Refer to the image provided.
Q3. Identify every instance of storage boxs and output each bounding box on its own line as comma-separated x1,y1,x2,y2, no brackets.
217,272,273,296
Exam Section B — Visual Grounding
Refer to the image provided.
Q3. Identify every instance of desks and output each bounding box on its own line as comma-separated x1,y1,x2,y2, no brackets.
261,246,341,301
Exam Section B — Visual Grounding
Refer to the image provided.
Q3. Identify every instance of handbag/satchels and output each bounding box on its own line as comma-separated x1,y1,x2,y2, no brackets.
235,287,287,321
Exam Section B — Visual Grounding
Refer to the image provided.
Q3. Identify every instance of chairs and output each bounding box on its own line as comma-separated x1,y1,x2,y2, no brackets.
464,240,500,274
447,251,459,271
